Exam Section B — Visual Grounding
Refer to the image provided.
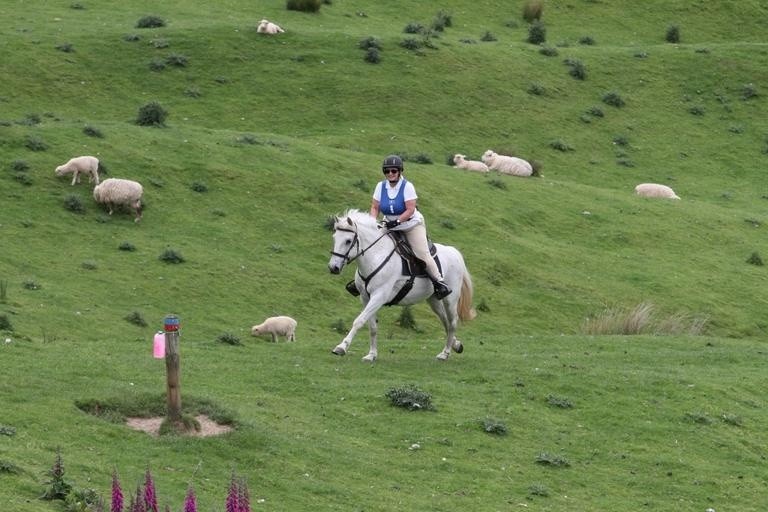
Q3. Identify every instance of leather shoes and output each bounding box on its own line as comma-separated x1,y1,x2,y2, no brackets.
347,282,359,296
434,281,447,297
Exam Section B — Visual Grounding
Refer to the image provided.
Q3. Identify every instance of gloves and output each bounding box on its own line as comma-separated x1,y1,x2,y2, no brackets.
384,220,400,229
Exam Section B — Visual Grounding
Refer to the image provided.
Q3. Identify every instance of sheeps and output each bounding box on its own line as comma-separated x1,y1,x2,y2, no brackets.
256,19,285,34
452,154,490,173
251,316,298,343
481,149,534,177
54,155,100,186
92,178,144,224
633,183,682,201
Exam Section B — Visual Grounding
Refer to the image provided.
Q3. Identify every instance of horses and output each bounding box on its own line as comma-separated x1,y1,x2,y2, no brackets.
327,207,474,363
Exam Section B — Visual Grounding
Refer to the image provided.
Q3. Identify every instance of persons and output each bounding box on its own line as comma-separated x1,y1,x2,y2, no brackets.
348,155,449,299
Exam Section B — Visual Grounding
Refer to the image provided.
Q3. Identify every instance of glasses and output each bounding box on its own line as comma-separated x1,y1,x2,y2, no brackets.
384,170,397,174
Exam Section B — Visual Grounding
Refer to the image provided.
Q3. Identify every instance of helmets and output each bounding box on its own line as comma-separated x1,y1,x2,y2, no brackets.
382,155,403,169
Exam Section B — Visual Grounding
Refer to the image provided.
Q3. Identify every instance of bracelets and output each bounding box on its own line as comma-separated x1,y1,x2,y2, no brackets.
396,218,401,225
